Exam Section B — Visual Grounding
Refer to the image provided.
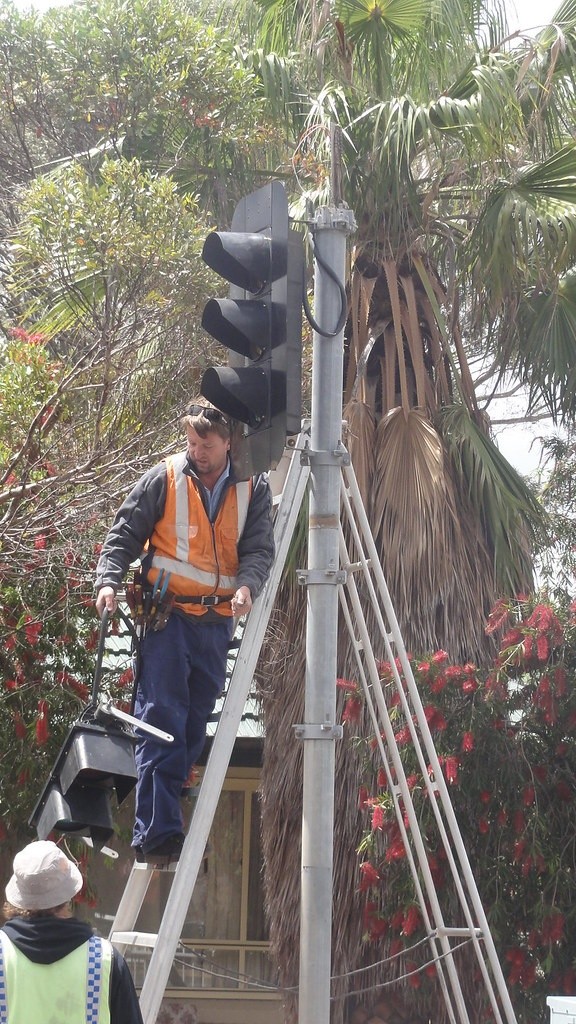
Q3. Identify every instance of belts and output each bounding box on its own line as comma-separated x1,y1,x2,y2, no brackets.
174,594,233,607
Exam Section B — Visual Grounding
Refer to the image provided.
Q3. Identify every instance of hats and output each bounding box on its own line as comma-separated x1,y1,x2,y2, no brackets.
5,840,84,910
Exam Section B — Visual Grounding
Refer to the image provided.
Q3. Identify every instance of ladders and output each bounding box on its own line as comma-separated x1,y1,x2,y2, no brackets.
108,414,517,1024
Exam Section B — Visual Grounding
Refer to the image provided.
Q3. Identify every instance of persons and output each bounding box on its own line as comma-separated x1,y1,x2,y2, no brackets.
92,399,277,866
0,838,145,1024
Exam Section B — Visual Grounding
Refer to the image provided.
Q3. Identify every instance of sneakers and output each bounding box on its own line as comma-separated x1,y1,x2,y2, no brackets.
143,831,208,871
134,847,145,864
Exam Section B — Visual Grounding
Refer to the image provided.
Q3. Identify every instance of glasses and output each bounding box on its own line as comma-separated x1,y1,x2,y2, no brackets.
188,404,226,421
